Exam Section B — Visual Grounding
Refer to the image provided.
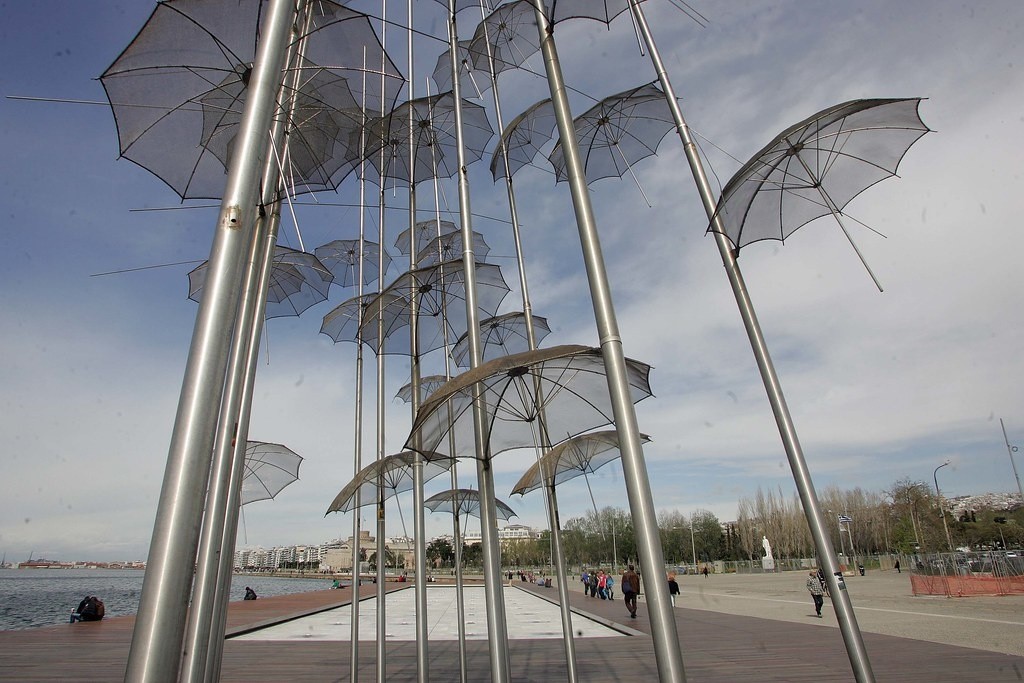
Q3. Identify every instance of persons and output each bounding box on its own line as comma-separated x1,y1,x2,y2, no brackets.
763,536,772,557
668,576,680,609
894,559,901,573
328,579,339,589
703,567,708,579
244,587,257,600
517,570,527,582
428,576,435,582
582,569,614,601
817,568,825,589
807,569,823,618
66,596,104,623
622,565,640,619
394,575,406,582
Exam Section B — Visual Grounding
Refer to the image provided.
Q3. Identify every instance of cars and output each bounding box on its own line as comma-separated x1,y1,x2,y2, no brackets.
923,549,1018,579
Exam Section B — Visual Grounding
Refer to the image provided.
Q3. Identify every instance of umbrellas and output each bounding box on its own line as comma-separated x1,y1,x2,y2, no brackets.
95,0,939,550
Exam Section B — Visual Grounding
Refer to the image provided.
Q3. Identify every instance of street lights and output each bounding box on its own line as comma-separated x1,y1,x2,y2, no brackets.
914,494,930,565
934,459,961,578
597,532,622,575
907,481,923,554
672,521,698,574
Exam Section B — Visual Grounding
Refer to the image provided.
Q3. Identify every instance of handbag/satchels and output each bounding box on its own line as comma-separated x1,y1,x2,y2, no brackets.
623,581,632,594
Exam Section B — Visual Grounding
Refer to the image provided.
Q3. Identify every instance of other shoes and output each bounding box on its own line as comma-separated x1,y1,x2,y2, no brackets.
818,610,822,618
631,611,636,618
